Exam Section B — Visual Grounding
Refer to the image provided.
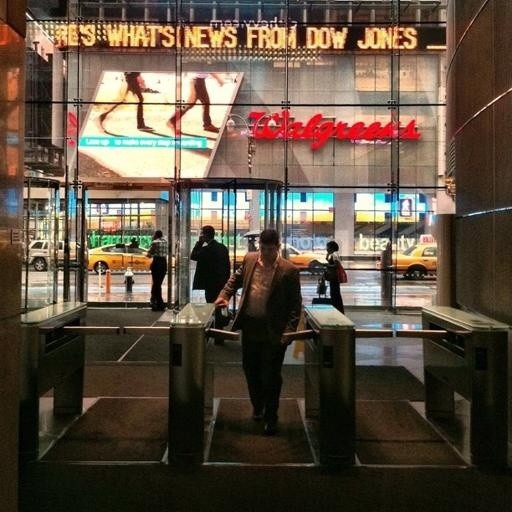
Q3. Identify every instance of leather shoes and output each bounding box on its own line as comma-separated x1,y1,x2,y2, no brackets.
264,420,275,433
253,407,263,420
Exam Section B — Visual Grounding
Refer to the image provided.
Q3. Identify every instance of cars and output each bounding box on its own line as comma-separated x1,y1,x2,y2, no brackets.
84,244,178,274
229,243,322,276
376,234,438,281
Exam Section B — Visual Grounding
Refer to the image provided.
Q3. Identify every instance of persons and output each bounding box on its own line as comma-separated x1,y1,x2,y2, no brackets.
245,238,257,252
214,228,302,437
146,230,167,312
164,73,226,135
188,226,231,346
93,72,155,134
322,241,349,316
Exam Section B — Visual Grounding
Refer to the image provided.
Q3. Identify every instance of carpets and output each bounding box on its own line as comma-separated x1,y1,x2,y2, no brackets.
21,307,512,511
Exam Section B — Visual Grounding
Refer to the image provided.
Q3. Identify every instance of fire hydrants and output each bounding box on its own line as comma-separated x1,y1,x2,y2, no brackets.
121,266,136,292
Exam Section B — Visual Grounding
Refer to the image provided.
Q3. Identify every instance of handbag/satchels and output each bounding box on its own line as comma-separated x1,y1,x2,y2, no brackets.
335,260,347,283
316,279,327,294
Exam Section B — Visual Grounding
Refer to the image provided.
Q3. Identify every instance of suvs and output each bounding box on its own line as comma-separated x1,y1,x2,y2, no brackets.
23,240,94,274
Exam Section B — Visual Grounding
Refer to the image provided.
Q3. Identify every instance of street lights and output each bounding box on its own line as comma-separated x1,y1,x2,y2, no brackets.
225,110,279,178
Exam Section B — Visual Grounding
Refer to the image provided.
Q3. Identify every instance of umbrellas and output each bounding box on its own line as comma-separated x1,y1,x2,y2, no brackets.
244,229,263,237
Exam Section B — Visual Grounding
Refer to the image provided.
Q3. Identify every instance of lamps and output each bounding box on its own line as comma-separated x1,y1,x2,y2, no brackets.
226,110,277,175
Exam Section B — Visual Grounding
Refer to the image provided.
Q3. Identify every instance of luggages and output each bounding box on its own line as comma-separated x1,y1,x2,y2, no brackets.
312,276,332,304
